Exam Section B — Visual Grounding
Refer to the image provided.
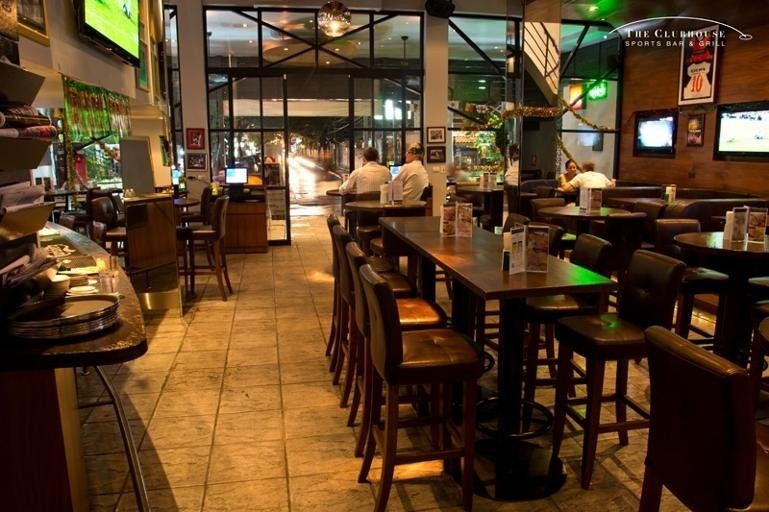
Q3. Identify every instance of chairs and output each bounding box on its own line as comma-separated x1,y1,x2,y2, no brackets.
59,181,233,301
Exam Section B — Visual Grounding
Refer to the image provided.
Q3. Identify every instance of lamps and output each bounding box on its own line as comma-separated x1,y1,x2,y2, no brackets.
317,0,351,37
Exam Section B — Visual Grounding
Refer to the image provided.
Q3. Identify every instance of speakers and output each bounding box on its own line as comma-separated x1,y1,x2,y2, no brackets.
425,0,455,19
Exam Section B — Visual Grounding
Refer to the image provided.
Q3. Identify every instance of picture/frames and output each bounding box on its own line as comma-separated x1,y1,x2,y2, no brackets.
185,149,207,172
686,113,705,147
427,146,446,163
186,128,204,149
676,24,720,106
427,127,446,144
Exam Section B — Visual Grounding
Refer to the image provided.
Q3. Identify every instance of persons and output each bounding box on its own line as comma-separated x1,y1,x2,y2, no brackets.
447,164,470,183
563,160,611,209
506,144,520,185
557,158,578,187
214,168,226,184
337,146,394,195
394,141,430,202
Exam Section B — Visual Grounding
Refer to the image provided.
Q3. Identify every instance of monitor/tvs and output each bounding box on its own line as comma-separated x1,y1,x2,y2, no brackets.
74,0,141,69
634,111,675,155
715,105,769,157
388,165,401,180
225,167,248,184
